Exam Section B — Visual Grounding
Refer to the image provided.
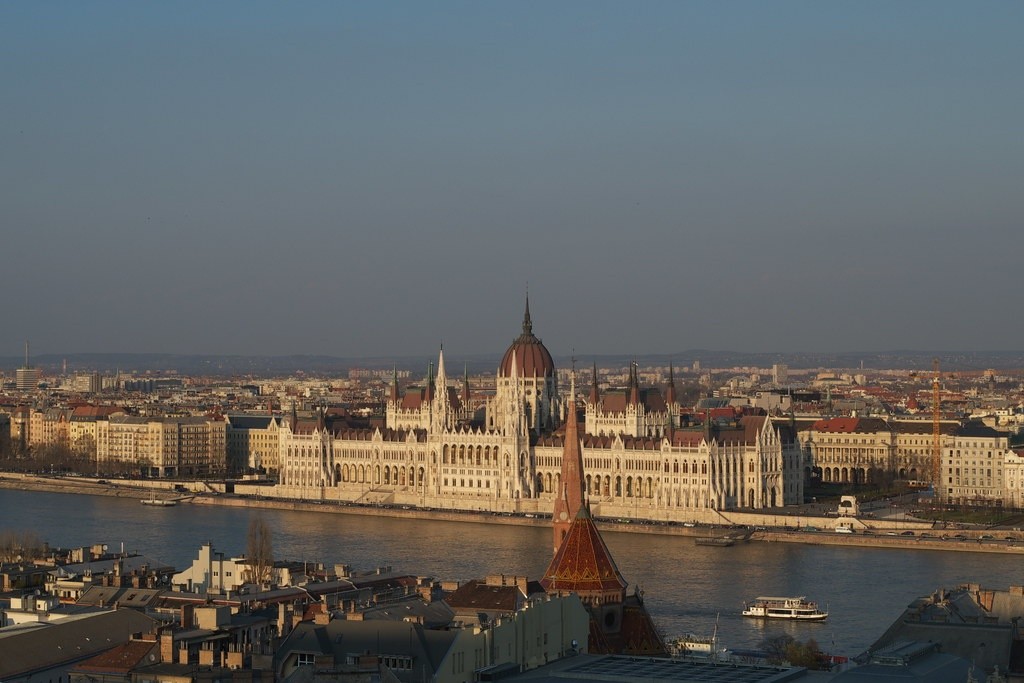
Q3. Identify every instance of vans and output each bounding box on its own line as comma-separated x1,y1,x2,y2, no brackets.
836,526,853,534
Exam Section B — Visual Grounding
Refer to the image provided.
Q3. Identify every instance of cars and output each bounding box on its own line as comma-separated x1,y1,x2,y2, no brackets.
863,531,1017,540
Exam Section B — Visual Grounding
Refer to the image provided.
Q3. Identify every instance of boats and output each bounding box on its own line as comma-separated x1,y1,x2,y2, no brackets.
741,594,831,619
695,537,736,547
141,498,177,506
